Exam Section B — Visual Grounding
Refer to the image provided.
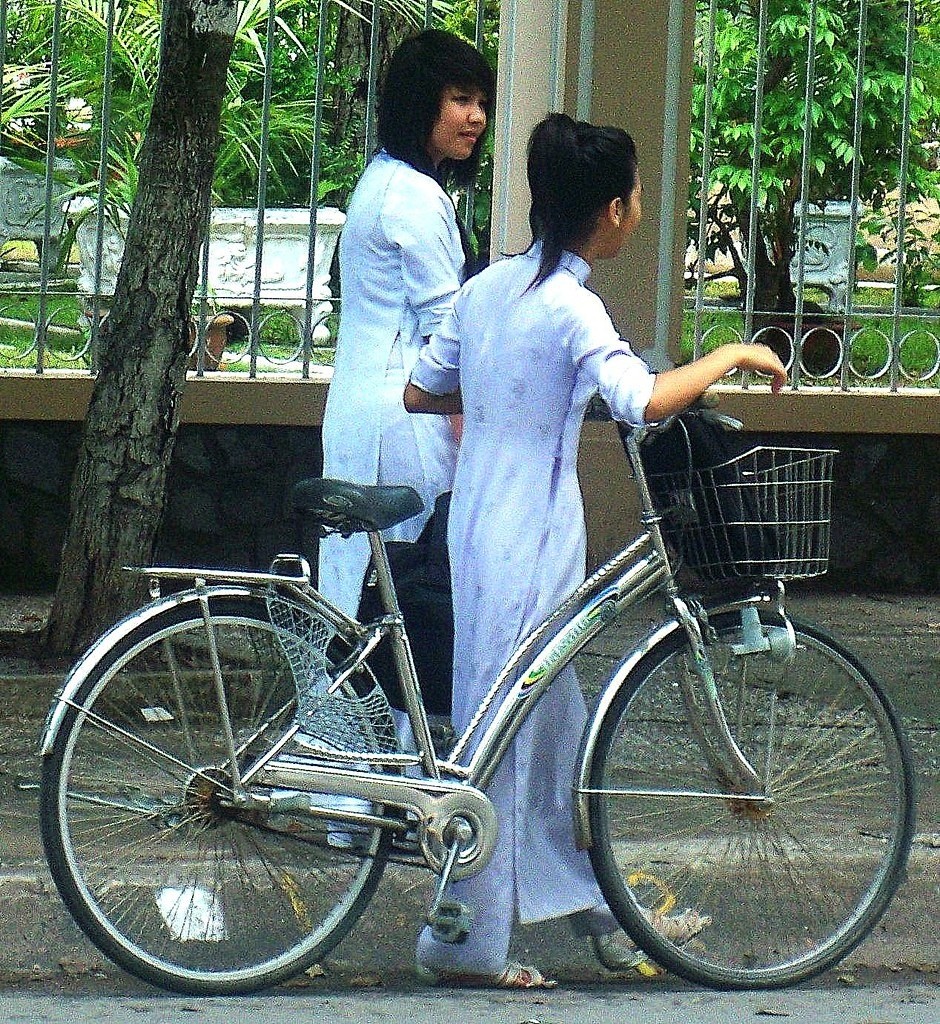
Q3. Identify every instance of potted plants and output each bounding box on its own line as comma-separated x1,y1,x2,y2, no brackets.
0,0,350,373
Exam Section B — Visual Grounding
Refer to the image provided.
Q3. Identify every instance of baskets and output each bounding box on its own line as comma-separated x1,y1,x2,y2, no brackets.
645,446,840,581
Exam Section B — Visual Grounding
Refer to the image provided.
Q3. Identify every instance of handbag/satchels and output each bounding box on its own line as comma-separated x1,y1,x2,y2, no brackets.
618,408,785,579
325,493,453,716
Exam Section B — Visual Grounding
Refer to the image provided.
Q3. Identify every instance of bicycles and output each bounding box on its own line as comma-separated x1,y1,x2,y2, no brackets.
36,369,916,997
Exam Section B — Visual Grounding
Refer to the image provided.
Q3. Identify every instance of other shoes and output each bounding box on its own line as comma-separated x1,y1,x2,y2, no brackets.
328,823,366,848
392,830,419,850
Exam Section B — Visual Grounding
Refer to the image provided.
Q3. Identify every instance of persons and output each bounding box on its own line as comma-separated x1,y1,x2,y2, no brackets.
310,29,494,850
403,113,786,989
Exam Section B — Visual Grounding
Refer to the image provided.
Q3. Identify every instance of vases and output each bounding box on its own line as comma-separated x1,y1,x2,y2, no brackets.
777,320,864,376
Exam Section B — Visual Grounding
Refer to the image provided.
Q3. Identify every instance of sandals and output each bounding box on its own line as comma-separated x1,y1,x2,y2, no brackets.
419,960,555,989
591,908,712,972
